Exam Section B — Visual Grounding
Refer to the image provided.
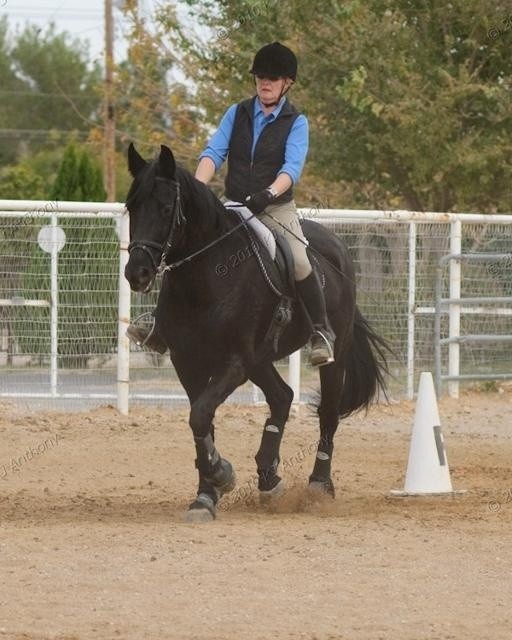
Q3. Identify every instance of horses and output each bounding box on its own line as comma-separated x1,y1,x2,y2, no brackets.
122,141,401,528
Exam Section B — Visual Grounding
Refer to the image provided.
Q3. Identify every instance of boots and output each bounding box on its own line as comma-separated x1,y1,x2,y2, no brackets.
127,322,167,354
296,268,337,363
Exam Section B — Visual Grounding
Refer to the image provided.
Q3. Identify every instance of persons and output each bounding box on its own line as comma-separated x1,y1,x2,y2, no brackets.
122,40,340,368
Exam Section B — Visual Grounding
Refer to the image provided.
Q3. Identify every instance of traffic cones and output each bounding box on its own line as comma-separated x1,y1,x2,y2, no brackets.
389,369,470,497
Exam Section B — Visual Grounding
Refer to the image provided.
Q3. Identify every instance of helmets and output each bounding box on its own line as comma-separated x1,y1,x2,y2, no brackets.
251,42,298,77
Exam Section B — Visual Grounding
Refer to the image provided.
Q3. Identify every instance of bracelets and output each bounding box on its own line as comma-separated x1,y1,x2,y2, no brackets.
265,186,279,201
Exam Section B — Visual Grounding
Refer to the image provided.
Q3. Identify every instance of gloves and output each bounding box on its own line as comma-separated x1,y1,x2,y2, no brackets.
245,189,272,214
